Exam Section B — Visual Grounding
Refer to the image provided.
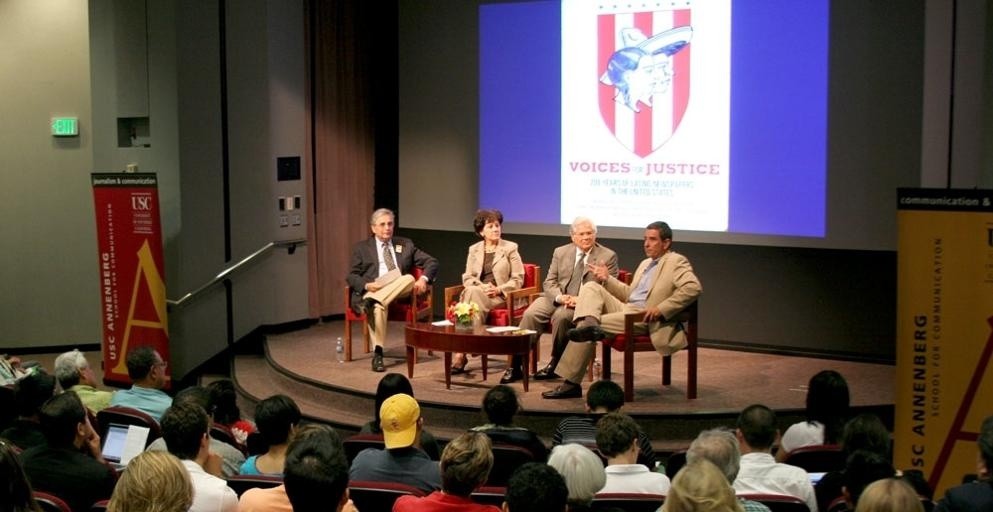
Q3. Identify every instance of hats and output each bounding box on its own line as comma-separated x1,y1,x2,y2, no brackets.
379,393,422,451
600,26,693,86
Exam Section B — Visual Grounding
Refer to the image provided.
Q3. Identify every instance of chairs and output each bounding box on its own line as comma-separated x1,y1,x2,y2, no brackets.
344,269,434,363
446,264,541,367
530,270,632,381
602,297,699,401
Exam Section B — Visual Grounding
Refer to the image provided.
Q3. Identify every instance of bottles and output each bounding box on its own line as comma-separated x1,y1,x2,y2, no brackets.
593,358,601,380
335,337,344,363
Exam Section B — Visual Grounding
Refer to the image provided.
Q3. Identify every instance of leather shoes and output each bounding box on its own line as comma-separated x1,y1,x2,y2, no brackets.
533,366,557,381
451,358,468,375
501,367,522,384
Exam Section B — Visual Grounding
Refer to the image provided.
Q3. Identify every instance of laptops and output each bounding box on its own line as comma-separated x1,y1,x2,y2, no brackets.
99,423,129,472
807,470,833,487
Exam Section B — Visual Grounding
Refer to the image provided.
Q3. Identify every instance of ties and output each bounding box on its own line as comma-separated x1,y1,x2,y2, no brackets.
566,252,586,296
383,242,396,272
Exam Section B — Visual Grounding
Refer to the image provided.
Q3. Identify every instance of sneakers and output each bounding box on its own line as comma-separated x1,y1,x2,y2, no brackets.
567,326,606,342
371,353,384,372
542,383,583,399
353,303,365,317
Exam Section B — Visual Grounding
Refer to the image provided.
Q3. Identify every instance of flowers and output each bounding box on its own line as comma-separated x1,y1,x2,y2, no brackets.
445,301,478,324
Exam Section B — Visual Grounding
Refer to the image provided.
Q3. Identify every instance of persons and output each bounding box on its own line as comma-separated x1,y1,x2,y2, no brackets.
347,209,440,371
447,207,526,374
500,216,620,384
542,220,703,400
0,346,992,512
